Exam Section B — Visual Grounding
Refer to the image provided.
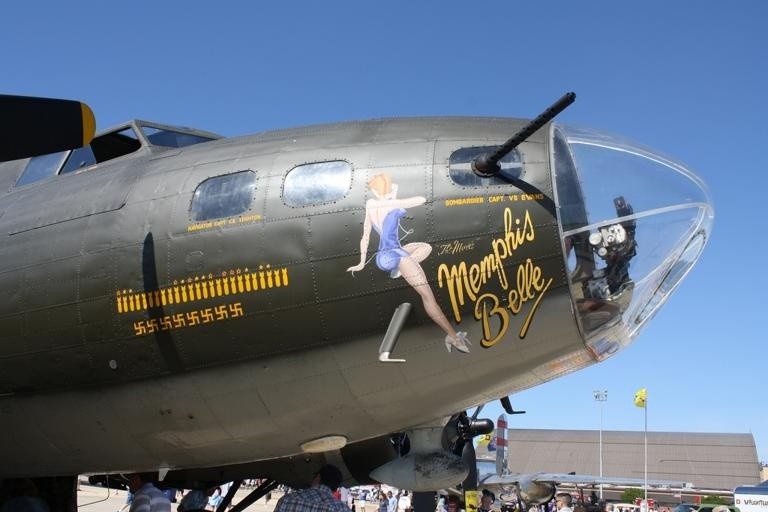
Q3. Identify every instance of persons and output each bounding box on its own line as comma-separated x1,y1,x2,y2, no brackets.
345,172,473,354
116,463,600,512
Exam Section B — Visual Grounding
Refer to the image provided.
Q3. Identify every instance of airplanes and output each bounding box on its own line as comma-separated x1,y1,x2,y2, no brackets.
0,92,714,512
478,473,685,512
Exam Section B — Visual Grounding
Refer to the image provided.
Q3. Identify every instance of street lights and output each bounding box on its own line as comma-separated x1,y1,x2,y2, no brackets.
592,388,607,499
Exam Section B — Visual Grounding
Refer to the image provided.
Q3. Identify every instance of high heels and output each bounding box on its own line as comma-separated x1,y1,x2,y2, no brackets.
389,267,402,280
442,331,472,356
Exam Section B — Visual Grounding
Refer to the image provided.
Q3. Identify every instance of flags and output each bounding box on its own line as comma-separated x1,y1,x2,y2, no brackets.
632,388,647,407
